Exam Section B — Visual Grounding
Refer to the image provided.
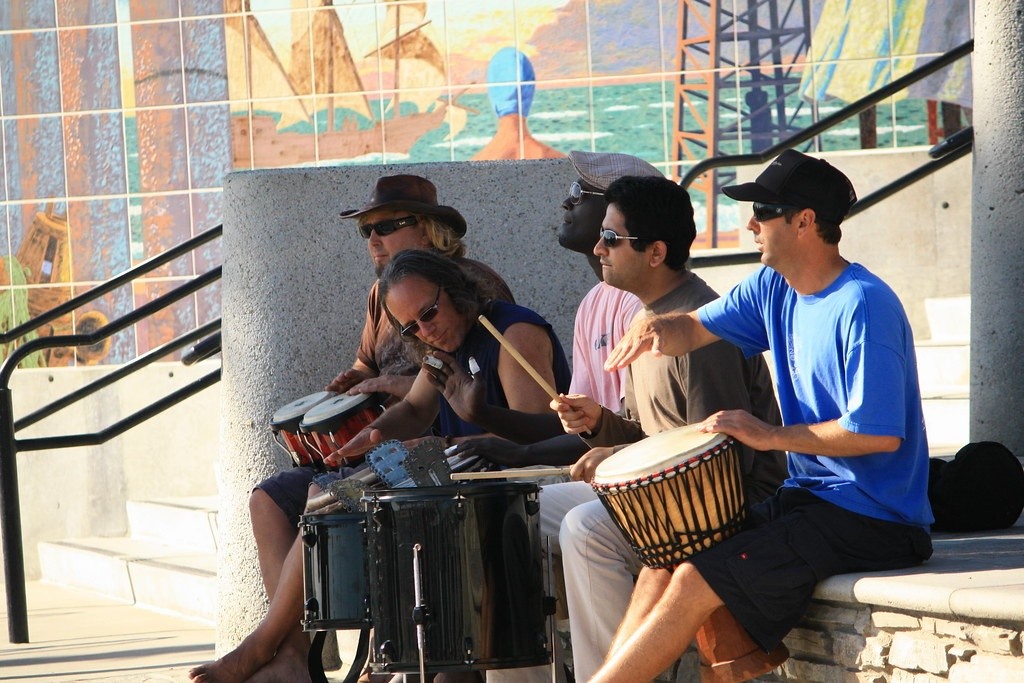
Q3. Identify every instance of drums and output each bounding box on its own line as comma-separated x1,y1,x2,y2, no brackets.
361,480,554,677
269,390,346,473
591,420,749,570
300,389,385,473
296,513,373,634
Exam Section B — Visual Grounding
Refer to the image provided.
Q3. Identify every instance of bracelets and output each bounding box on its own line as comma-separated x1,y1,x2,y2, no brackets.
365,426,383,434
445,434,452,449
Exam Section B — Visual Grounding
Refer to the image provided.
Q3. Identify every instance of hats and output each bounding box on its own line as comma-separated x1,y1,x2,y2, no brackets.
338,174,467,240
568,151,667,197
722,148,858,227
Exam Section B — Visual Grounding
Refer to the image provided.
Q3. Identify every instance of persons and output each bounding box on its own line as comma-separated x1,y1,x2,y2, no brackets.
586,150,933,683
324,250,571,683
423,151,786,683
187,175,517,683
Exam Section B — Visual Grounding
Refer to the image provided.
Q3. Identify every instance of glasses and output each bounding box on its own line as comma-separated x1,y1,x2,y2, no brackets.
752,201,801,221
568,181,607,205
358,214,422,239
600,228,639,247
401,284,442,337
